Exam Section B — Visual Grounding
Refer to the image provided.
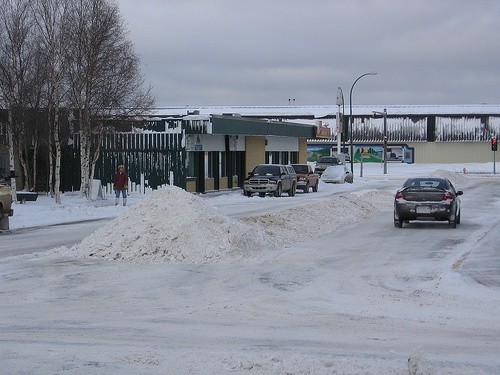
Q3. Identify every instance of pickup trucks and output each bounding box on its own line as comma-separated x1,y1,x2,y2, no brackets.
291,164,319,193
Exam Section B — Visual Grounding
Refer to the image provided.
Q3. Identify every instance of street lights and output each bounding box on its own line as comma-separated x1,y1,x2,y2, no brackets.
350,72,377,173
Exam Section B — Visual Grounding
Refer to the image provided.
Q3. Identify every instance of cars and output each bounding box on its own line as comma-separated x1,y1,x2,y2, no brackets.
320,166,353,186
393,177,463,229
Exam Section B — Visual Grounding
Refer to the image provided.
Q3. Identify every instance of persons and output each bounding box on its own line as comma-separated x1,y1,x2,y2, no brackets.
113,165,128,206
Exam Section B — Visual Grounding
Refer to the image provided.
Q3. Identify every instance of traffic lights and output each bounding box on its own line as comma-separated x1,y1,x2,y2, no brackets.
491,138,497,151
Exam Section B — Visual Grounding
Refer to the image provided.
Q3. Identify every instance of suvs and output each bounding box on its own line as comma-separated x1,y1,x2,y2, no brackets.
315,157,343,175
242,164,297,197
